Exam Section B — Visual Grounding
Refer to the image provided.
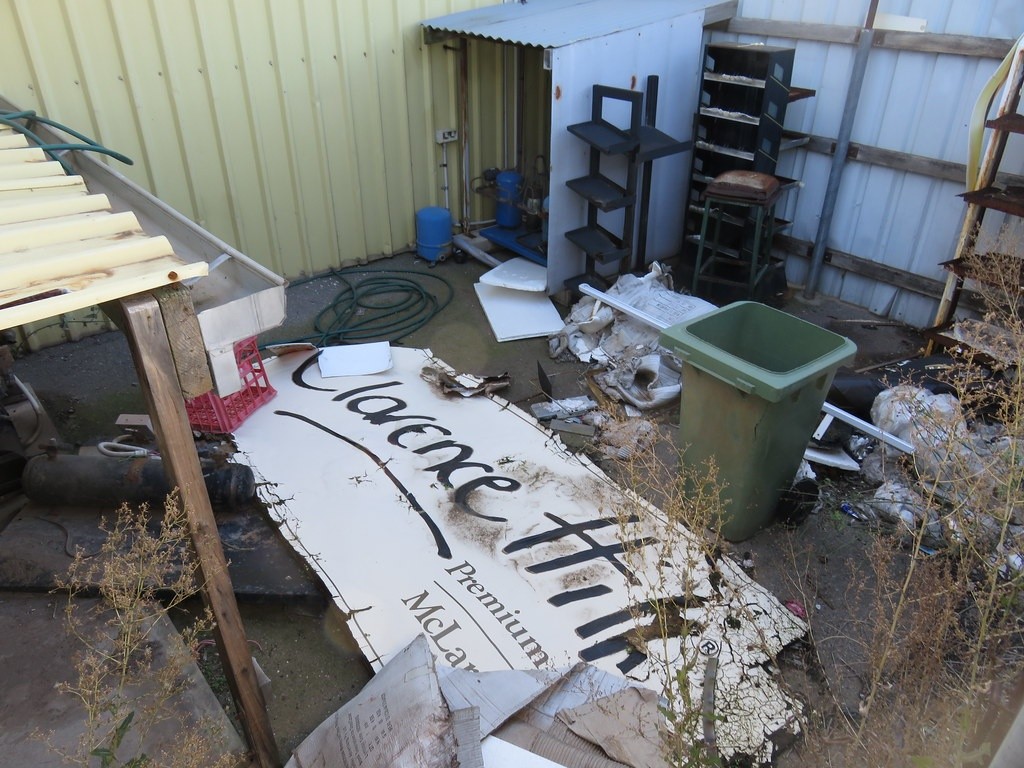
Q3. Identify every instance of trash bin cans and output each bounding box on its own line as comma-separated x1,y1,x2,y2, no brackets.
658,300,858,543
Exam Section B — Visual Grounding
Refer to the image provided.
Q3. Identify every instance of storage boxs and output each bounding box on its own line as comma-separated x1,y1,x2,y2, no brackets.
185,335,278,434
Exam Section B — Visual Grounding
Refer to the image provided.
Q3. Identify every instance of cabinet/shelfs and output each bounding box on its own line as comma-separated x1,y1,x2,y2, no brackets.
921,37,1024,374
676,41,816,304
561,83,693,297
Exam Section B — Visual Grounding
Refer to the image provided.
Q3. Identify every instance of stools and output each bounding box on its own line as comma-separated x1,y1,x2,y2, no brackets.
691,169,784,301
